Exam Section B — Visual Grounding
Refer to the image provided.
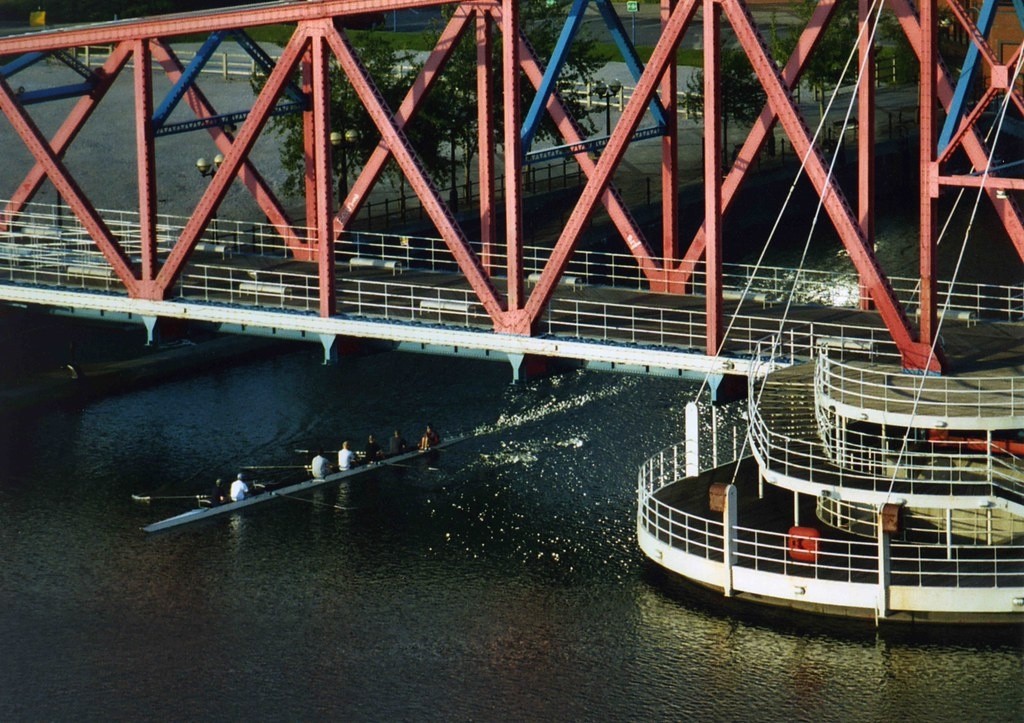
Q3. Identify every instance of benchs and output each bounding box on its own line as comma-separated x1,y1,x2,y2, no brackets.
237,282,294,302
814,339,879,360
194,244,234,261
349,258,403,276
65,266,120,285
419,301,478,318
915,309,978,327
526,274,582,293
723,291,775,310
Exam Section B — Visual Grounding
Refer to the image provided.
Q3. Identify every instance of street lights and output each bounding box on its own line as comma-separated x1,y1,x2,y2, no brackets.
595,79,622,136
330,130,360,210
196,154,227,246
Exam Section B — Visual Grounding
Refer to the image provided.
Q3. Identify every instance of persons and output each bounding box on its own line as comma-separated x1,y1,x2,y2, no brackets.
418,423,439,451
213,473,249,505
389,429,407,455
312,435,386,480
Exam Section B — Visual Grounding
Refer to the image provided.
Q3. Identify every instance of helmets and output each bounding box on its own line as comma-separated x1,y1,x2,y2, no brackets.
237,472,248,482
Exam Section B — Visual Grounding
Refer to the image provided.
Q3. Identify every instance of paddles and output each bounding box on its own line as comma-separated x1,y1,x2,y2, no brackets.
129,493,215,503
237,464,312,471
377,461,439,471
293,448,362,457
271,492,360,512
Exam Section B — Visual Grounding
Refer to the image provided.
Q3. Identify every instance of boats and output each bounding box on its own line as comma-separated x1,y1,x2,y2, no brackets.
137,433,475,535
